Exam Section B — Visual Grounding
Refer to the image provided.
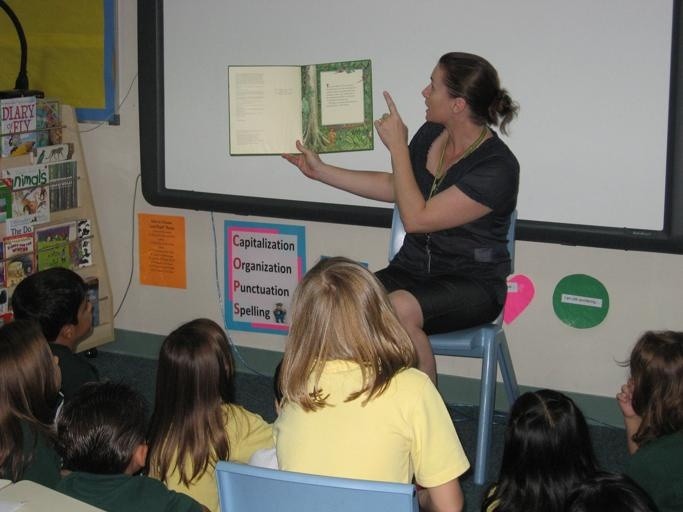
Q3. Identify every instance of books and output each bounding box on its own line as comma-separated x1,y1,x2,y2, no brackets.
225,58,373,159
0,94,103,327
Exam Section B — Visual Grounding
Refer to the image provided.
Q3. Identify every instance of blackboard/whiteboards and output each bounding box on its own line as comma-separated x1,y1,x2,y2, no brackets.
137,0,683,253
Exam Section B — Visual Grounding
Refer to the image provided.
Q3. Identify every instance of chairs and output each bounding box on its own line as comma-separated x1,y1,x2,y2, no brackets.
382,202,521,487
216,459,419,512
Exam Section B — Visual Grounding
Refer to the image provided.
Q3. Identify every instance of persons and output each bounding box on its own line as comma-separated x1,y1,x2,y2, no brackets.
10,267,101,406
50,380,213,512
476,389,598,512
559,470,659,512
278,51,519,386
608,331,682,511
133,318,276,512
0,320,69,489
247,358,286,471
272,257,472,512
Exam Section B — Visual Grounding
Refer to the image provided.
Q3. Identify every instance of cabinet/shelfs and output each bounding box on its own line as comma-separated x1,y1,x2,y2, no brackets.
1,101,115,363
